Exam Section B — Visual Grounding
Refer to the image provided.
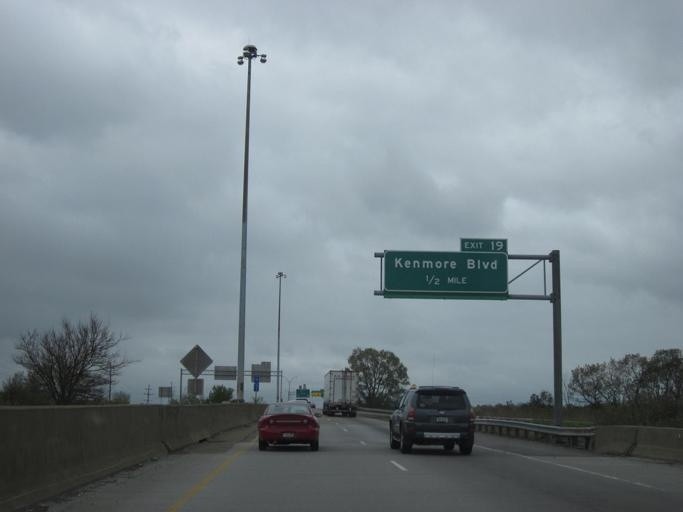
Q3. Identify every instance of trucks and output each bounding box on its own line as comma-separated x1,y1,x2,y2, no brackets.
321,369,359,418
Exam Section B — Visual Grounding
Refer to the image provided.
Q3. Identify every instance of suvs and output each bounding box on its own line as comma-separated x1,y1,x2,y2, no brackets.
388,383,475,456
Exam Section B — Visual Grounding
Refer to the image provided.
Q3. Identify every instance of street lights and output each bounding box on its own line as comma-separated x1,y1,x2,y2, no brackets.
231,40,265,406
272,270,288,405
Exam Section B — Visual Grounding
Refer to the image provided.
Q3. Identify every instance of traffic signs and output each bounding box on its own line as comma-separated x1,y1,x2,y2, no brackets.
459,238,508,252
383,249,508,295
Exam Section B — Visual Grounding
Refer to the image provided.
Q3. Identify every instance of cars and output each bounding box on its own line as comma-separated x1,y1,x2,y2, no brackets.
257,400,319,451
